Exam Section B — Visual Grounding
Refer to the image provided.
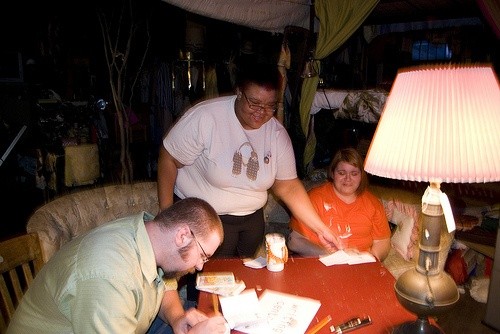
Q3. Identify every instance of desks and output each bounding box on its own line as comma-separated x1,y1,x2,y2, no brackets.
196,258,444,334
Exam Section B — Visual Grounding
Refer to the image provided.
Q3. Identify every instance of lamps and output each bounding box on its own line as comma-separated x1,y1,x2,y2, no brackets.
363,64,500,334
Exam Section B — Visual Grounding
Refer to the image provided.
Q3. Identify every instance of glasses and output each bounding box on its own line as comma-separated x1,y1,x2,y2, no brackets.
191,230,210,263
242,91,278,113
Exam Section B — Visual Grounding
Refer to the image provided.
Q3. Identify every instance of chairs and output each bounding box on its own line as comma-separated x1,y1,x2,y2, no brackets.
0,232,45,334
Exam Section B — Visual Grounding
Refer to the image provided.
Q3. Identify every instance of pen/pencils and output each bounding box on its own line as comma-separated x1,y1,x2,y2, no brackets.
305,314,332,334
337,317,371,334
290,255,295,263
212,294,219,317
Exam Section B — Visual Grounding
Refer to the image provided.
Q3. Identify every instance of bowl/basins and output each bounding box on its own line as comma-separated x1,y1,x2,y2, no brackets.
481,210,499,232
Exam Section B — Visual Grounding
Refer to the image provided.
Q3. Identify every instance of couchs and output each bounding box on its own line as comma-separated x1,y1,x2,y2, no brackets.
28,182,454,280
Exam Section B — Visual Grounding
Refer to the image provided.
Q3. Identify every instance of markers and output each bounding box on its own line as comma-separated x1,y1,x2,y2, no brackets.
330,318,361,333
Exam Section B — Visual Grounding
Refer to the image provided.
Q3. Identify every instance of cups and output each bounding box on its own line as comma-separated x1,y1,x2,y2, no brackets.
265,233,285,273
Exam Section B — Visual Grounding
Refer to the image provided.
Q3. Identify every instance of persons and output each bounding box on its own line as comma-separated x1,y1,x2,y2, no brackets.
288,147,391,262
157,67,341,304
4,198,227,334
